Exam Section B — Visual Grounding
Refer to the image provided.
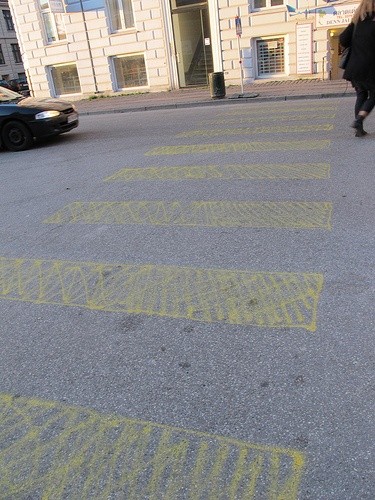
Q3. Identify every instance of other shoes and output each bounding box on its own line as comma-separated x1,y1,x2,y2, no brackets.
354,128,367,137
353,119,362,129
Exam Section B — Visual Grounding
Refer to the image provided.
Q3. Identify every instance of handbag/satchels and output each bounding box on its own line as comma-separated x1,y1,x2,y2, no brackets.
338,47,351,69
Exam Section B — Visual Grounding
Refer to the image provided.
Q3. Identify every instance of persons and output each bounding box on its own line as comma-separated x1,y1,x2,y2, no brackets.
339,0,375,135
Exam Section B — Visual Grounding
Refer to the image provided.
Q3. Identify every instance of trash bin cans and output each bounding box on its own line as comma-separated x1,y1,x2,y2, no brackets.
209,72,226,98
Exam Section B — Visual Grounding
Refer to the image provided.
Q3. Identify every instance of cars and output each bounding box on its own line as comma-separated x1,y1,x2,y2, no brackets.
7,79,30,96
0,86,79,152
